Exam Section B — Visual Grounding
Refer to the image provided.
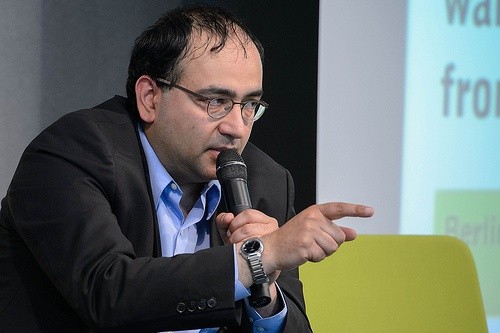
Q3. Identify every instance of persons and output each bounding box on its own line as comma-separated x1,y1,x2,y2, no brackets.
0,7,375,333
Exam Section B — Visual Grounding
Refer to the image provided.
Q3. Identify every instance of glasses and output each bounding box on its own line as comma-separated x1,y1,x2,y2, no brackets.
154,76,269,122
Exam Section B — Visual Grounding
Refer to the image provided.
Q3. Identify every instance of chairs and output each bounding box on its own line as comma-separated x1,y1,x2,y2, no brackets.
296,233,488,333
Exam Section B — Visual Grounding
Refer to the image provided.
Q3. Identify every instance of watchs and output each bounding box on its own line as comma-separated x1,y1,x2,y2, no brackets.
240,237,269,284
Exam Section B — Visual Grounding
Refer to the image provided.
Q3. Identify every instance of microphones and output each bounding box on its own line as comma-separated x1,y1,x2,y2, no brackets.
216,150,270,309
